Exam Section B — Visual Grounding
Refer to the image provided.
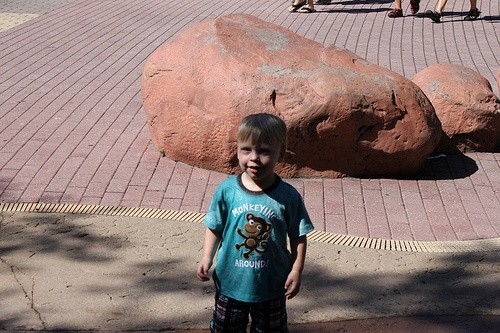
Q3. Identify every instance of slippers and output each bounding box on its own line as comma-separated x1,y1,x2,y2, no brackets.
289,1,316,12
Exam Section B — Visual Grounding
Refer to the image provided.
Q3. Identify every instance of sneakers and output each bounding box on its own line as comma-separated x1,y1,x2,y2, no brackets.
388,7,403,17
426,9,441,21
464,8,481,20
410,0,420,13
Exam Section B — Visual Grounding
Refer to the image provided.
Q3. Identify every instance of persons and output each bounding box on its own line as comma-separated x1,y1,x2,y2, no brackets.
288,0,316,13
196,113,314,333
388,0,421,18
426,0,481,23
